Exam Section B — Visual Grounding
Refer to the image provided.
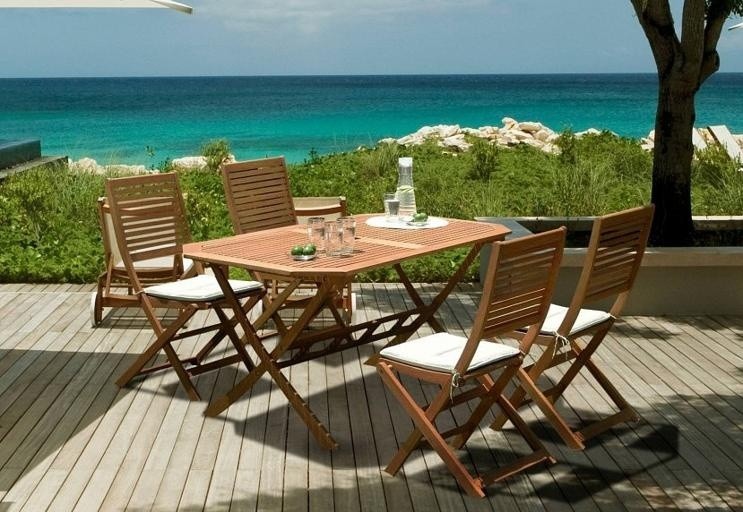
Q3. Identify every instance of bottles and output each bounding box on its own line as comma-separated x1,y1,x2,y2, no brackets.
394,157,417,222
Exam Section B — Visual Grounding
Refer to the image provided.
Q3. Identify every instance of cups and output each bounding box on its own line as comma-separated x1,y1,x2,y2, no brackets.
384,200,400,217
305,216,356,257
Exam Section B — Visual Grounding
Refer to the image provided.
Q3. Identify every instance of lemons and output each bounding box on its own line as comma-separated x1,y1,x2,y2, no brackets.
291,244,316,256
414,212,428,222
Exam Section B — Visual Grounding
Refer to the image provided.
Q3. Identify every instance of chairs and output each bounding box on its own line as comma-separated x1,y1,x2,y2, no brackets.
100,169,269,403
465,202,653,455
93,192,205,331
259,193,357,333
220,157,357,358
361,224,570,498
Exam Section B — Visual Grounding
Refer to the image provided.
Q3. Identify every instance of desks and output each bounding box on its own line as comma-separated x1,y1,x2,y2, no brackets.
177,209,517,452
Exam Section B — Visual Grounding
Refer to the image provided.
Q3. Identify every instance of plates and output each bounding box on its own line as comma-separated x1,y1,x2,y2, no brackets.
285,251,317,261
